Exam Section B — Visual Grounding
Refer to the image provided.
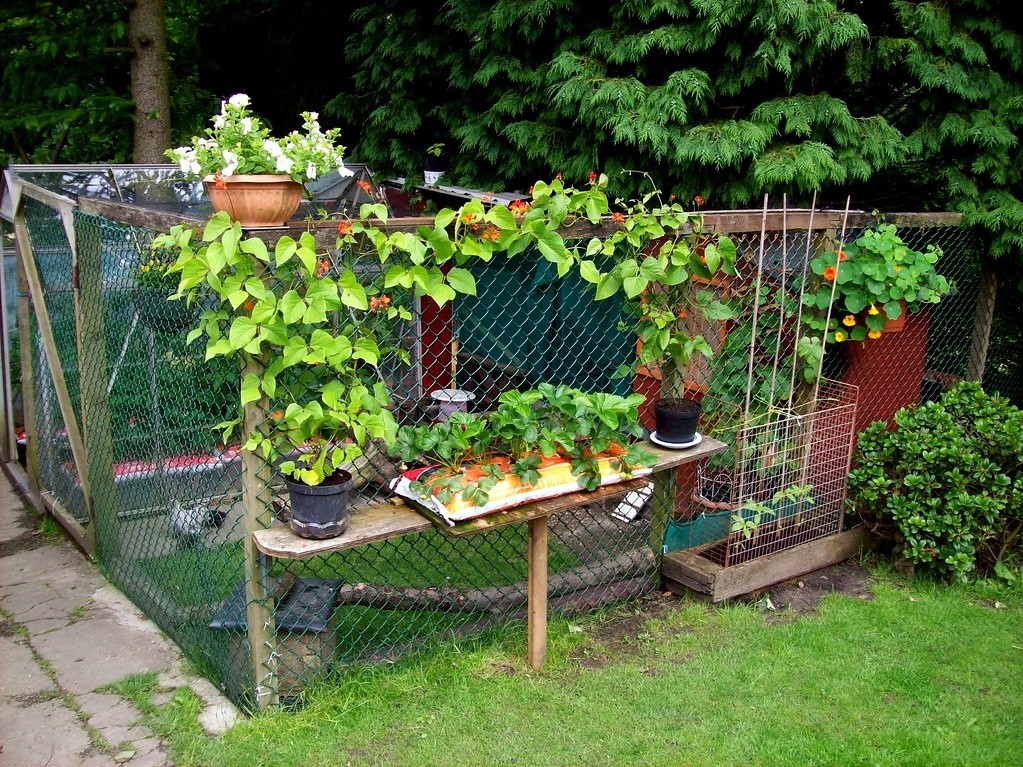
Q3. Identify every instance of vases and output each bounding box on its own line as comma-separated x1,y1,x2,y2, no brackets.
137,290,196,333
205,174,306,228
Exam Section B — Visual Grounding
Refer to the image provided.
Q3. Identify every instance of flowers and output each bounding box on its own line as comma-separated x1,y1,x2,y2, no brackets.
163,93,356,182
132,251,177,291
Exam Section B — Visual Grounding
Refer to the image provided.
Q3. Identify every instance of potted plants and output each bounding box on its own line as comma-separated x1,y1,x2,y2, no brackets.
153,169,956,539
